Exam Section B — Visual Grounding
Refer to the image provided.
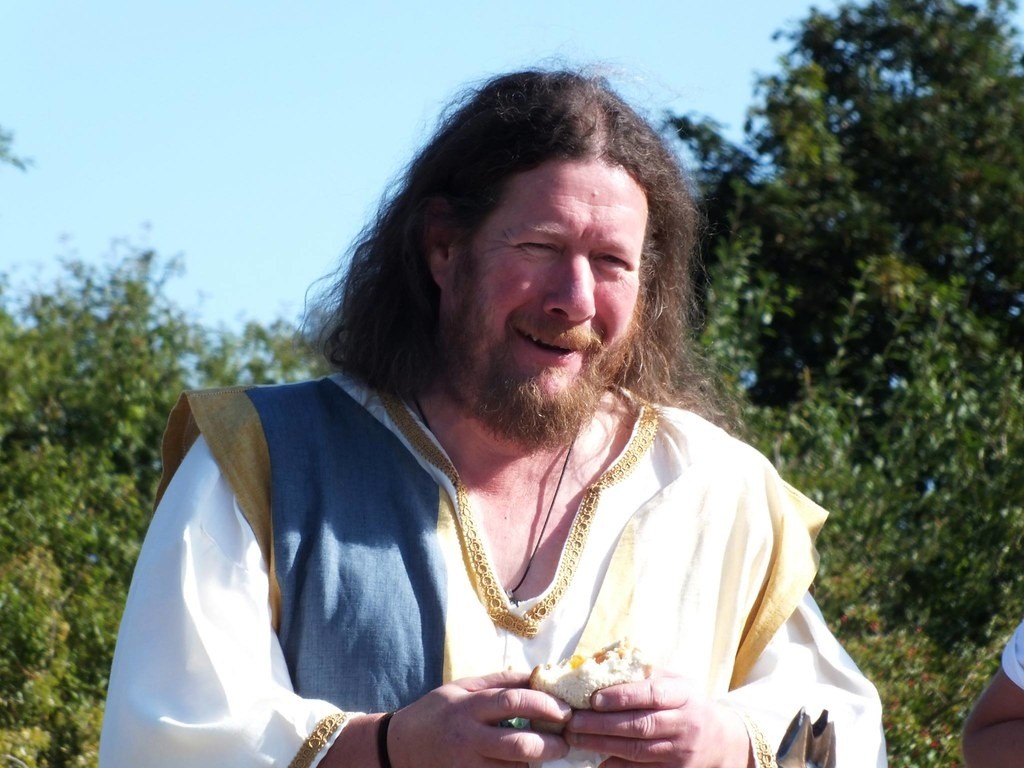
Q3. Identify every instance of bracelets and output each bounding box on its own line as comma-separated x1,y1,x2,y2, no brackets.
376,710,397,768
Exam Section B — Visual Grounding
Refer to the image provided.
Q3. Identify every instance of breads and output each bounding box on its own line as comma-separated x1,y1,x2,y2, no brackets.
525,638,651,734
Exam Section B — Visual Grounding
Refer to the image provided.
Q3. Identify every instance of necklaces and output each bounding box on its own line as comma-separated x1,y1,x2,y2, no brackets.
409,388,577,607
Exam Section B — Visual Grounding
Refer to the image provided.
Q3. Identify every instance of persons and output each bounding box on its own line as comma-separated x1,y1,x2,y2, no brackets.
95,69,889,768
962,617,1024,768
